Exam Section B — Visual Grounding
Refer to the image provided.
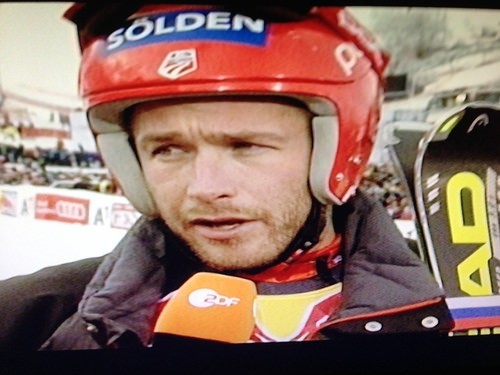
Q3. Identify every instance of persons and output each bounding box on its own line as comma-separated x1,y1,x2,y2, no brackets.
41,0,456,352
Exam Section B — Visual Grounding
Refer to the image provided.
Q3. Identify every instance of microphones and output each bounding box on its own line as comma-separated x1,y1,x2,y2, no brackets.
152,271,258,346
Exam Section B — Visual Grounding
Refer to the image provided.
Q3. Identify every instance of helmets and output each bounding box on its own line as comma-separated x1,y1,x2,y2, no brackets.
62,0,389,219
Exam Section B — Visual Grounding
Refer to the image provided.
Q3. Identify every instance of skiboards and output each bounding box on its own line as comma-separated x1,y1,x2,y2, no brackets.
385,102,500,297
